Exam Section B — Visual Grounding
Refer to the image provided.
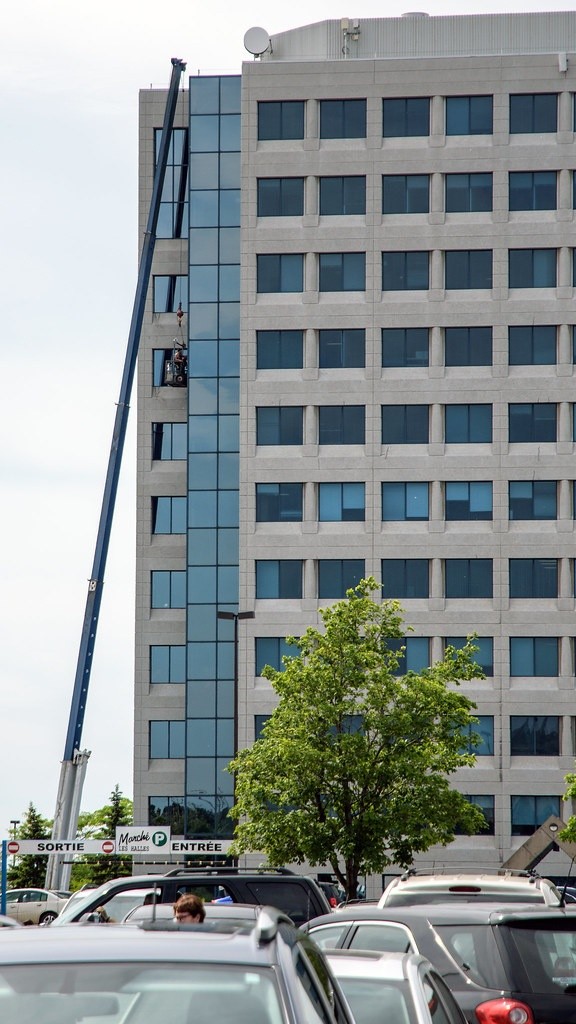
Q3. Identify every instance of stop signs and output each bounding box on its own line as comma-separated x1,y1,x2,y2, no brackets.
102,841,114,853
8,842,19,853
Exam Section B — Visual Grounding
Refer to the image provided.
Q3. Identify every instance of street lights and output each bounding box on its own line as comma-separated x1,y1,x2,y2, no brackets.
217,611,255,873
10,820,20,866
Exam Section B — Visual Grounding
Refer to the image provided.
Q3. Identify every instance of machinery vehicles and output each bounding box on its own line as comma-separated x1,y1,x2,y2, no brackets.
42,54,189,890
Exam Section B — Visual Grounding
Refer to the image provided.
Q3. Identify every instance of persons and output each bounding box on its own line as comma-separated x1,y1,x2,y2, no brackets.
174,892,206,925
97,893,160,923
173,348,186,370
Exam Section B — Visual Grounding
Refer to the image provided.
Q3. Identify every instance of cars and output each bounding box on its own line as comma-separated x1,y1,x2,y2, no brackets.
0,886,576,1023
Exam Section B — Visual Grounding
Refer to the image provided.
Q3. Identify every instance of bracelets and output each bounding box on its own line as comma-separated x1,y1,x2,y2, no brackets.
106,916,110,923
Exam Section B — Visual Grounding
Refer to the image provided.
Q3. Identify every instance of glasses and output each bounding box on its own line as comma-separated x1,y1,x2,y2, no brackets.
176,914,193,922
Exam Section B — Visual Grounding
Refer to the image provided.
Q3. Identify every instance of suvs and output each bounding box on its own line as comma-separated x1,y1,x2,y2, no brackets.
51,866,334,930
378,867,576,986
